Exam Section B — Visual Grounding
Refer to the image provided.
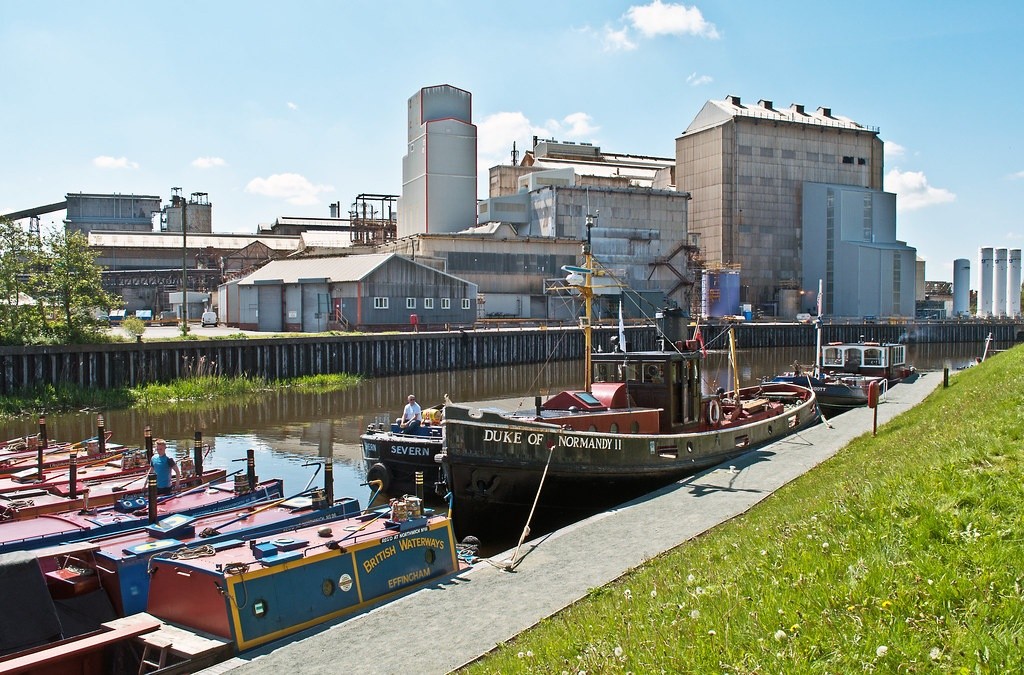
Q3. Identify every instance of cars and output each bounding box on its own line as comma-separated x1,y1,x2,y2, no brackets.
862,315,875,324
201,312,217,327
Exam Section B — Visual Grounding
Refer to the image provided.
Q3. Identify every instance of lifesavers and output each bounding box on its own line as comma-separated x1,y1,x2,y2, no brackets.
707,400,720,425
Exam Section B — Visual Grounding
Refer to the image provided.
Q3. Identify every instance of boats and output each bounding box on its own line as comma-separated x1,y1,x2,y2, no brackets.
768,336,912,409
1,412,482,675
442,224,821,498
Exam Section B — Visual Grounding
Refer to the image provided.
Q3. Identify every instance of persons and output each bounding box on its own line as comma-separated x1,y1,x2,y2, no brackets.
141,439,180,495
395,394,422,434
790,360,800,376
